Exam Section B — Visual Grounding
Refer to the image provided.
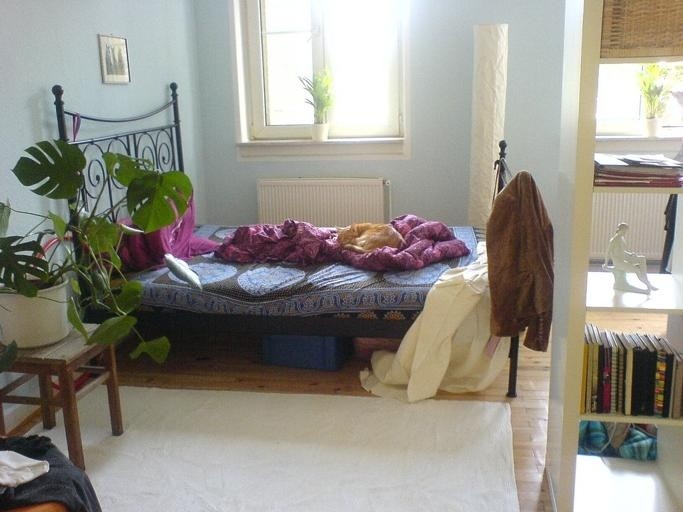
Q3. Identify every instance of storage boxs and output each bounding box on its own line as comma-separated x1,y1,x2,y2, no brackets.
270,334,345,371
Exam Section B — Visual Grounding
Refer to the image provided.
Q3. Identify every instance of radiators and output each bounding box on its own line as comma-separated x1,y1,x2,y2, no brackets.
257,177,392,228
590,190,672,262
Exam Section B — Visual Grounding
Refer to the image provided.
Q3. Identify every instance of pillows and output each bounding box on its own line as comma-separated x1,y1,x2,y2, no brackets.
96,188,220,270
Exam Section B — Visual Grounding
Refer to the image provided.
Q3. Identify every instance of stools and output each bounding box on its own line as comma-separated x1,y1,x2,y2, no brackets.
0,322,124,472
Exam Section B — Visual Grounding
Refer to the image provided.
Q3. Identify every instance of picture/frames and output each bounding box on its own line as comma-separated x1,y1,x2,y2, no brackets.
98,34,130,84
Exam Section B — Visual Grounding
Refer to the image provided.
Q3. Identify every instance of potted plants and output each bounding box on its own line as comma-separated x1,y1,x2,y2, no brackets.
0,140,192,373
638,61,674,140
300,66,329,140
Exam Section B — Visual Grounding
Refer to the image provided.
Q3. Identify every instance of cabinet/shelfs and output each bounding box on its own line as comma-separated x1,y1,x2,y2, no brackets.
543,2,683,512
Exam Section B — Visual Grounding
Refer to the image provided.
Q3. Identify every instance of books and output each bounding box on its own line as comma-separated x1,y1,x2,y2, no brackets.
580,324,682,420
593,152,683,187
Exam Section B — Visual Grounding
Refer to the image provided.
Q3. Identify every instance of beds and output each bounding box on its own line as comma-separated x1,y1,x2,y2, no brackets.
53,82,518,396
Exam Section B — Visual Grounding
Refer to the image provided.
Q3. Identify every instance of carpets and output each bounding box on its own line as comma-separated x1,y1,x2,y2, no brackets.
22,383,519,512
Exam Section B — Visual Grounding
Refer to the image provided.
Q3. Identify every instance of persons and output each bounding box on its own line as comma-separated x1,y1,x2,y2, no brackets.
601,223,659,292
